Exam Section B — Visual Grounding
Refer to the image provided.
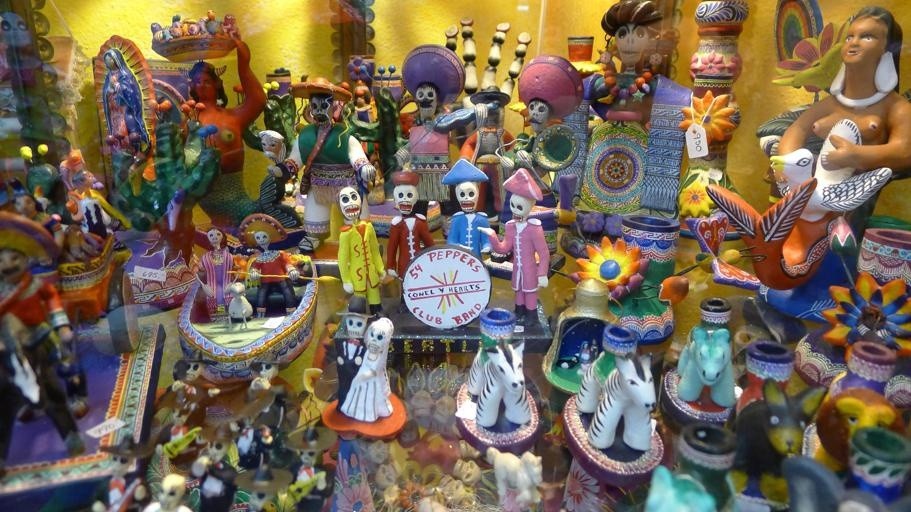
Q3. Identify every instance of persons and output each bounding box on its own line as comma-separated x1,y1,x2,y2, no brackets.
0,1,910,512
